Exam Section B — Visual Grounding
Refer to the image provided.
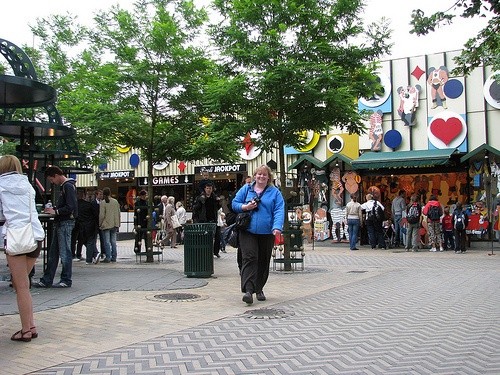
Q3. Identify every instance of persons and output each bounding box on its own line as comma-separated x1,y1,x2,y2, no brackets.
0,155,45,342
345,190,469,254
134,189,186,252
121,204,129,212
192,182,228,258
71,187,120,264
33,167,77,289
226,165,285,304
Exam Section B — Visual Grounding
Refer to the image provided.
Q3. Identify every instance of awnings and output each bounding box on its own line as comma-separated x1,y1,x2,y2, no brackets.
350,148,457,170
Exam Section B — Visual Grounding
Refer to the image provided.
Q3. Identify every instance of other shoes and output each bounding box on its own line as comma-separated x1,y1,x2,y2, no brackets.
33,282,47,288
242,292,253,304
111,259,116,262
257,291,266,301
94,252,100,264
53,282,68,288
429,248,436,251
100,259,109,263
440,246,444,251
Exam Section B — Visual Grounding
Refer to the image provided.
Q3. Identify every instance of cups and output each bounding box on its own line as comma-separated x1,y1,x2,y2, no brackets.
36,204,42,214
45,204,50,213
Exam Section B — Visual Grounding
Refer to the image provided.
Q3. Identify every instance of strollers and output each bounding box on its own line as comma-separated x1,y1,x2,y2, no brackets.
381,214,400,250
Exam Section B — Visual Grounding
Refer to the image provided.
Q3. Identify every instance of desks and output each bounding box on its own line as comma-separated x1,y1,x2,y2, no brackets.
465,215,489,248
0,214,56,273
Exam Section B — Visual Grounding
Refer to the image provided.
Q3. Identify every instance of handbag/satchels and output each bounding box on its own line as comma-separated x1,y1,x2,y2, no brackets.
373,201,386,218
5,223,36,256
406,203,421,223
223,223,238,248
234,212,250,228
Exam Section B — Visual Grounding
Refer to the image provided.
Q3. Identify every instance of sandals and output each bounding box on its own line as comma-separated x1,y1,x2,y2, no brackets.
11,326,38,342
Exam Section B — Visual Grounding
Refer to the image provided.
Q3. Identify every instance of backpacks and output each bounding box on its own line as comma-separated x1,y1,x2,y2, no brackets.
443,216,452,230
427,205,440,221
454,211,465,230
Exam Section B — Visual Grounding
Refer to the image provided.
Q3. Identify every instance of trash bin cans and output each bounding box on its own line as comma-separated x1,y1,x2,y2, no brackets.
180,223,216,278
282,220,303,251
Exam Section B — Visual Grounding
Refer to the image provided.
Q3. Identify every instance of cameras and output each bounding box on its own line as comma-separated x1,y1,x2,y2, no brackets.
253,197,261,203
